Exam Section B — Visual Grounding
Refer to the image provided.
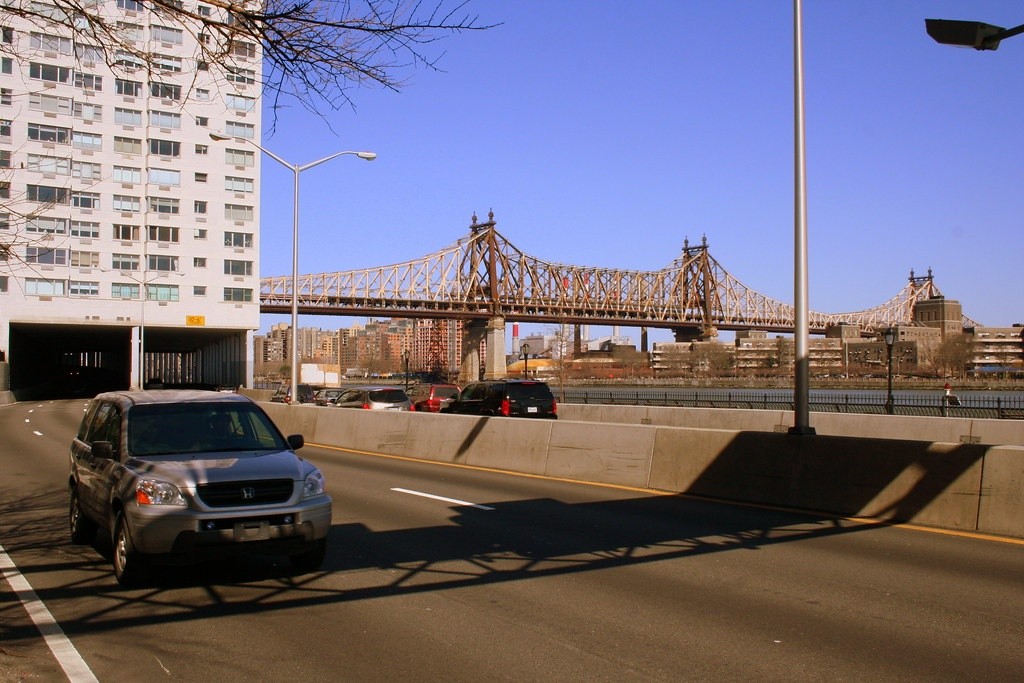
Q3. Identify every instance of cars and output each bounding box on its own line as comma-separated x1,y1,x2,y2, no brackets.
328,384,415,412
313,387,347,407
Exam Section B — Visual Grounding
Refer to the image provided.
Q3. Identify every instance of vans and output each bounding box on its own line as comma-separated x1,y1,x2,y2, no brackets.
405,382,462,415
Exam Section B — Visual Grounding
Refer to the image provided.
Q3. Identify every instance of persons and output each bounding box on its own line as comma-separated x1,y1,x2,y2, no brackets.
192,414,244,451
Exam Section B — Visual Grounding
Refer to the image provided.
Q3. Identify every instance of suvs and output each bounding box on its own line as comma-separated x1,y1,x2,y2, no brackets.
67,389,332,589
270,381,316,406
438,376,559,421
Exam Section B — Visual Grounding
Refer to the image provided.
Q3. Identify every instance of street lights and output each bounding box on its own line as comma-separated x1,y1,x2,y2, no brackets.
403,346,411,392
520,341,530,382
882,325,897,414
630,361,638,379
208,131,377,398
